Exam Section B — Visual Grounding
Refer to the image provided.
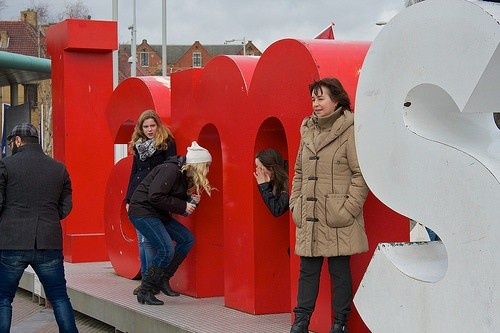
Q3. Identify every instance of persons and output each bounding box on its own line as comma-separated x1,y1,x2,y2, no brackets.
0,123,79,333
128,141,212,305
289,78,369,333
253,148,288,217
124,109,177,294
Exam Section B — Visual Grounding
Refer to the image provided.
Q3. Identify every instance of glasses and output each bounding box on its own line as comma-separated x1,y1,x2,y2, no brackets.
7,138,15,147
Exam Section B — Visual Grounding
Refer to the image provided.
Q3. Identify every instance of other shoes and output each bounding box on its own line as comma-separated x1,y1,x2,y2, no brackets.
133,283,160,295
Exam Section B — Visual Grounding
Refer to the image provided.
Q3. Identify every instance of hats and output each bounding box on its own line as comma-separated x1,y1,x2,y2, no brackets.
185,141,212,164
6,123,38,140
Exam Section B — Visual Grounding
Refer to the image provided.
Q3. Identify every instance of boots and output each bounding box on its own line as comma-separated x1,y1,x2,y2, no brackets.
136,266,164,305
289,312,312,333
330,318,349,333
156,270,180,297
